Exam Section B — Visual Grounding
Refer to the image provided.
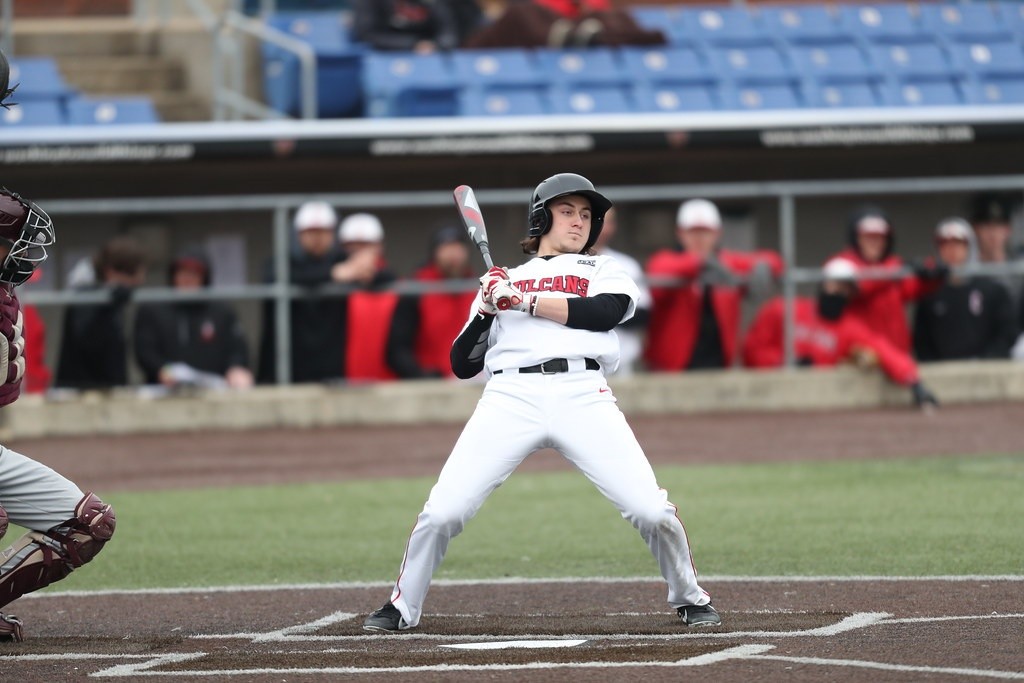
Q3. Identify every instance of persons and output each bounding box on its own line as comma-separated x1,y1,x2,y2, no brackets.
827,214,924,359
0,48,118,642
384,226,489,380
588,203,653,377
362,173,721,633
135,252,254,386
743,256,940,407
20,257,53,394
910,203,1024,361
57,234,146,389
349,0,666,51
647,198,783,373
254,200,402,385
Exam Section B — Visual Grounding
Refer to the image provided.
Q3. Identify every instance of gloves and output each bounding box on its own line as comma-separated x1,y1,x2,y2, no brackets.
487,282,539,318
478,266,509,320
910,384,942,412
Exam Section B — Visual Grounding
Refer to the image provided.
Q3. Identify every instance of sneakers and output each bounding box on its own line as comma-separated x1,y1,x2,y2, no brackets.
676,598,721,628
362,602,411,633
0,610,23,637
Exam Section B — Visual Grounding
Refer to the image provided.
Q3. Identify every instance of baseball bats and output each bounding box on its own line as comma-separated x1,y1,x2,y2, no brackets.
454,184,511,311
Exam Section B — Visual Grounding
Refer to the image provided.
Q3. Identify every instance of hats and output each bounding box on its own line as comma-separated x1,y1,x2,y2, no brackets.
430,222,473,264
676,198,723,231
291,197,338,232
930,215,972,241
846,202,892,235
168,249,212,289
820,256,859,286
339,212,385,246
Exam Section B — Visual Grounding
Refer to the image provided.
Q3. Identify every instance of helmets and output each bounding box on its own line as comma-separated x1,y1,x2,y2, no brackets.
528,173,613,255
0,188,56,299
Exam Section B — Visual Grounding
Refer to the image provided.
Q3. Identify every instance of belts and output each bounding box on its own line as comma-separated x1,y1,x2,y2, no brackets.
493,357,600,374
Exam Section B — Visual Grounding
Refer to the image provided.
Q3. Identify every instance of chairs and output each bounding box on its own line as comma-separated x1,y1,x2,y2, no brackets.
237,0,1024,117
0,55,161,124
